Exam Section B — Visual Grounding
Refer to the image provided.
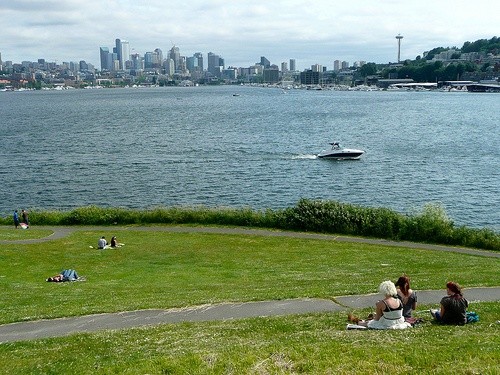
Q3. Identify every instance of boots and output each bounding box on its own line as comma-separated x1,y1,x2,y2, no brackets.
348,312,362,325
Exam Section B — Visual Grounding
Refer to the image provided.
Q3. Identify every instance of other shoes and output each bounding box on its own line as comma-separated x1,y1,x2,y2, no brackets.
430,309,437,320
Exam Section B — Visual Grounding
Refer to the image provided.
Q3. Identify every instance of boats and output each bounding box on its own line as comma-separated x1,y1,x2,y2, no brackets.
316,142,365,160
241,80,500,93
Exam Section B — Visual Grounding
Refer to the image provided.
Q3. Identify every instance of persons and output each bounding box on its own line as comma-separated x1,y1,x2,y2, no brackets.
14,210,19,228
390,276,417,320
431,282,469,326
111,236,116,247
97,235,108,249
21,210,29,229
347,280,406,329
